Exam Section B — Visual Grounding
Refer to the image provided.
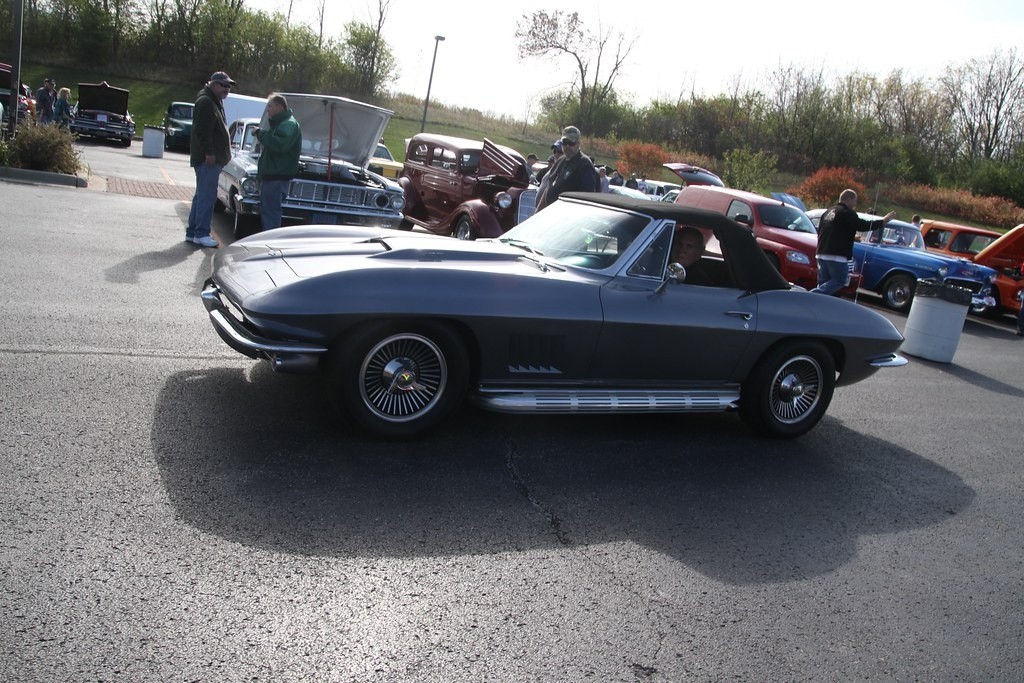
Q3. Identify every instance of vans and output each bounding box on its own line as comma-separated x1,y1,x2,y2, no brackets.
626,162,864,297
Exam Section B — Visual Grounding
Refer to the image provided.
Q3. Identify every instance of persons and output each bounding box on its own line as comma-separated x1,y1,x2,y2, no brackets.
638,175,648,193
35,78,58,125
625,173,639,190
905,215,921,242
185,71,236,246
53,87,75,129
524,126,600,213
671,226,721,288
810,188,896,296
610,169,623,186
599,166,609,193
250,95,302,232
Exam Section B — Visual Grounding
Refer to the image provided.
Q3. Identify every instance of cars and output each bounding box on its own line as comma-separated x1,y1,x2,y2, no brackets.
871,217,1024,329
216,92,407,239
789,208,1001,316
196,190,909,441
0,62,37,137
161,101,195,151
396,132,548,241
68,80,136,146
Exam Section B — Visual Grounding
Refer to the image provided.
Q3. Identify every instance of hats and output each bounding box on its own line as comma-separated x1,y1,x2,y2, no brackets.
209,71,237,87
526,152,541,161
561,126,582,144
547,155,556,163
550,138,563,152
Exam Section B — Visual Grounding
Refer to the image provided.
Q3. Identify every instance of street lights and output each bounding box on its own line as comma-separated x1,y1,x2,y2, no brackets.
420,35,445,133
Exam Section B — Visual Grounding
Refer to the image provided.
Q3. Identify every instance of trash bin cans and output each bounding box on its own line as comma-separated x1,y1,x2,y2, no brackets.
899,279,972,362
143,125,166,158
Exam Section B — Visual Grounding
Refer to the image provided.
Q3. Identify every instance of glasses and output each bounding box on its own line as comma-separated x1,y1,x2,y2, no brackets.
47,83,56,87
552,149,563,155
560,140,576,146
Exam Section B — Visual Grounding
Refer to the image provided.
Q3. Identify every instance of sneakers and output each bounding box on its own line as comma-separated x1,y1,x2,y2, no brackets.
185,235,215,242
192,235,220,247
